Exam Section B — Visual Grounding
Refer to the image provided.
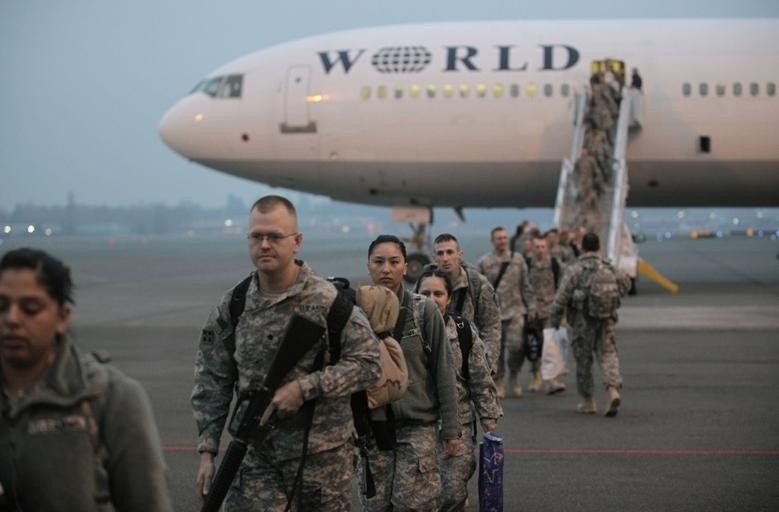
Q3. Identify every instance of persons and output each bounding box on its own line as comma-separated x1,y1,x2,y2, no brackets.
508,220,586,395
0,248,172,511
410,233,502,381
413,266,505,512
188,194,383,512
549,231,632,417
557,75,619,258
479,227,537,397
359,235,461,512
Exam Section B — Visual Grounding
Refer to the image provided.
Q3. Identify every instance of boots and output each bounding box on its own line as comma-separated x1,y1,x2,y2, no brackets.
576,394,597,414
603,386,621,416
495,370,566,398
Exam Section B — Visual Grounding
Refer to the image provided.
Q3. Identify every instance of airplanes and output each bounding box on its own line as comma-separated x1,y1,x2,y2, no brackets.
158,10,779,301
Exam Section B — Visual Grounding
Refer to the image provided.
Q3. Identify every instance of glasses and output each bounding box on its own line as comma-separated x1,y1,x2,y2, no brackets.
246,231,298,243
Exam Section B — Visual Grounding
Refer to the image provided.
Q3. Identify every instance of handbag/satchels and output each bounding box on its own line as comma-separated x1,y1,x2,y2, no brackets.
541,326,570,380
478,431,504,512
524,319,542,361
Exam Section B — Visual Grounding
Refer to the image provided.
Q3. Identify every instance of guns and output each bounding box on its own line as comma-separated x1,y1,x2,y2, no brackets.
199,308,325,511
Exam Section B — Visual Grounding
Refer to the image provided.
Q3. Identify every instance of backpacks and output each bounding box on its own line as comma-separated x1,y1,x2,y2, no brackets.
571,257,619,321
